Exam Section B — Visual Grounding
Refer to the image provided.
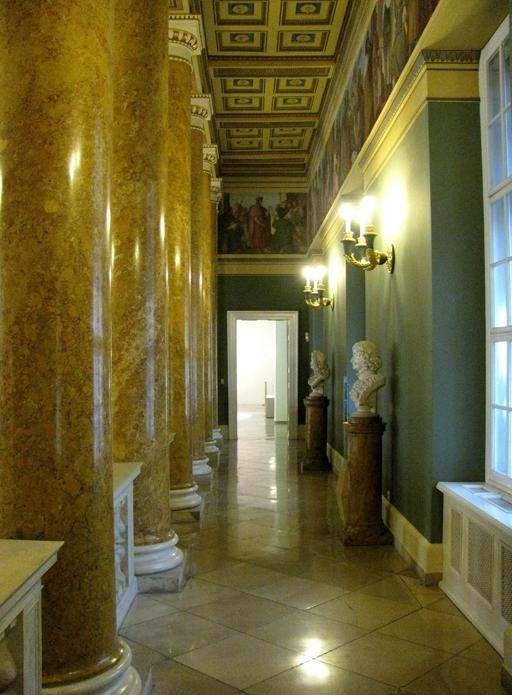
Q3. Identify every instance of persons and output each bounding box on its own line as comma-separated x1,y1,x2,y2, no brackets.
304,349,332,391
344,338,385,406
221,195,306,253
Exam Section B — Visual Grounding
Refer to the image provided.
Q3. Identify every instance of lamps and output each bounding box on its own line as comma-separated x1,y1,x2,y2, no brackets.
301,263,335,312
338,195,394,274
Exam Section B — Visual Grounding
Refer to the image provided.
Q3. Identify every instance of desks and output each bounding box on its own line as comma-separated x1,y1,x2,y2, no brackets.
110,461,141,634
1,540,64,695
